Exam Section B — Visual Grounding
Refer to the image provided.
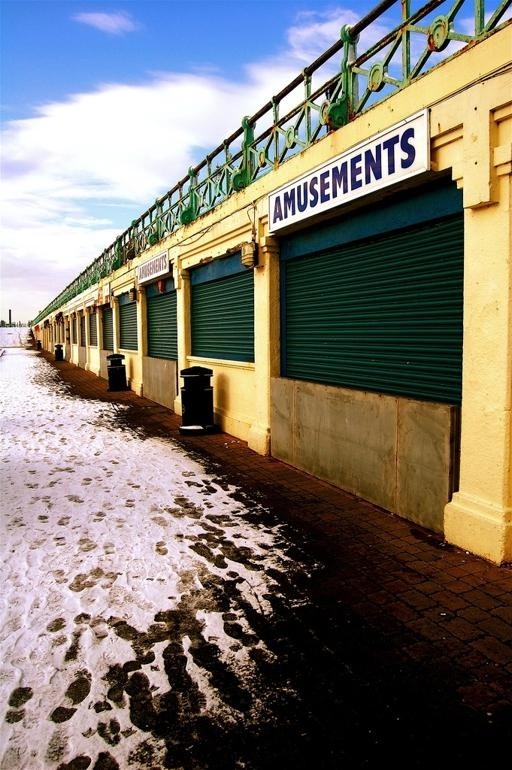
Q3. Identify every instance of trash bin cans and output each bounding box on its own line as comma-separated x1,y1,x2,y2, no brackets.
36,340,41,350
179,367,213,435
107,354,126,391
55,345,63,361
